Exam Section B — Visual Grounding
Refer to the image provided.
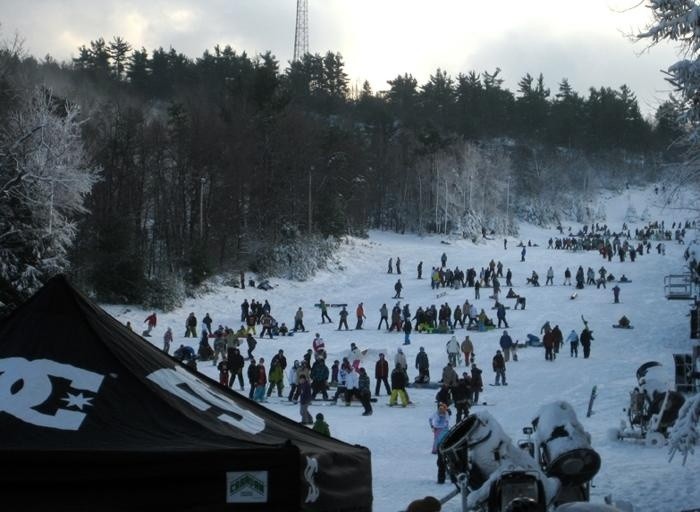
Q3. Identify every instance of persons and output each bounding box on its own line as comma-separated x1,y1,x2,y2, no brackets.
655,188,658,194
379,252,630,366
249,277,255,287
482,219,699,263
374,347,509,483
125,298,377,436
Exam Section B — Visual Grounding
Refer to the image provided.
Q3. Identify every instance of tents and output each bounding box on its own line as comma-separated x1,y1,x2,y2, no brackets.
0,275,374,511
